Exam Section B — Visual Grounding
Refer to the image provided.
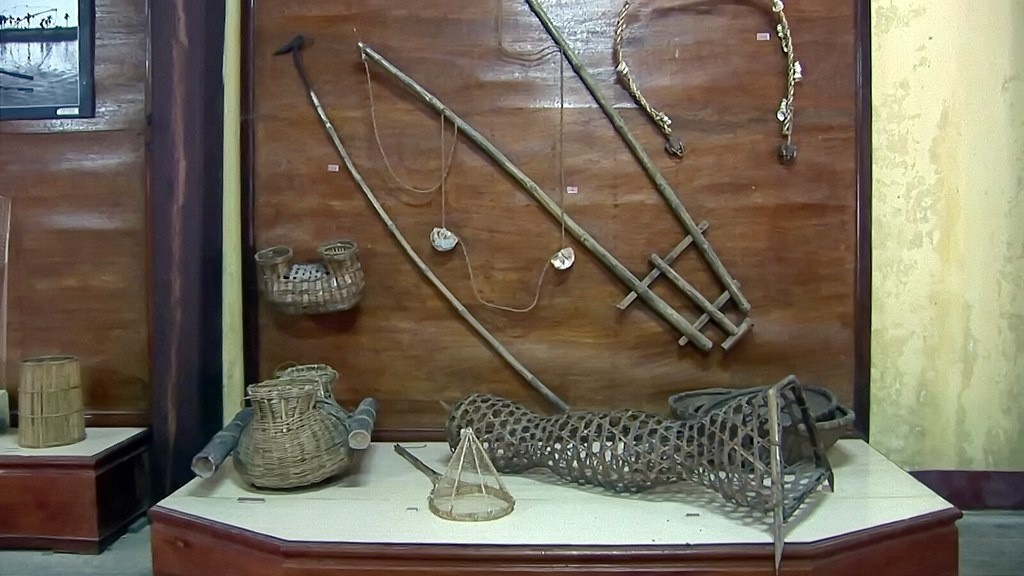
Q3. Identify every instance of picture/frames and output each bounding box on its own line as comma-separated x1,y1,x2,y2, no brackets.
0,0,95,118
